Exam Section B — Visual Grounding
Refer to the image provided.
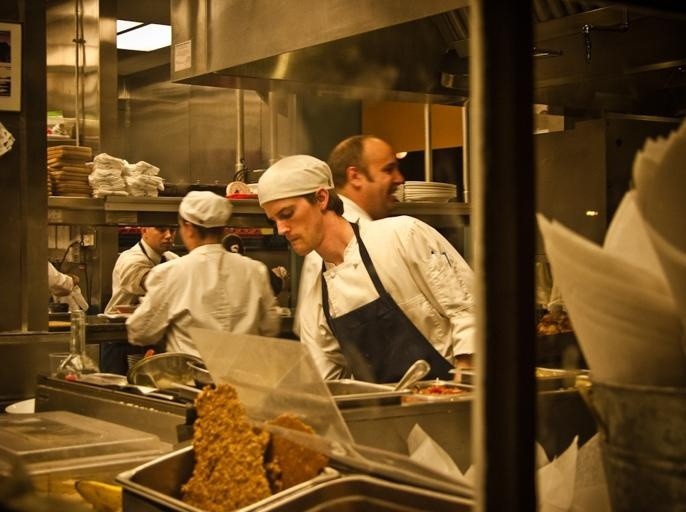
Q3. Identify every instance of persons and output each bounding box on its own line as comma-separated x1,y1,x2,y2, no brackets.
289,133,404,339
47,257,79,301
123,190,283,364
102,225,183,315
255,153,478,386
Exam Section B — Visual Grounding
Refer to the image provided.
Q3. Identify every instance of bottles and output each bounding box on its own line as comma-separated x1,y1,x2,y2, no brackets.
61,309,97,376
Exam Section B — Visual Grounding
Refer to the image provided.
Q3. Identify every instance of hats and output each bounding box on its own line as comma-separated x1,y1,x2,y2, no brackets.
178,191,232,228
256,155,334,208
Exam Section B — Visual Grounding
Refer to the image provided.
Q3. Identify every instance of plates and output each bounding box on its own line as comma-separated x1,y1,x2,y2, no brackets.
396,178,458,206
